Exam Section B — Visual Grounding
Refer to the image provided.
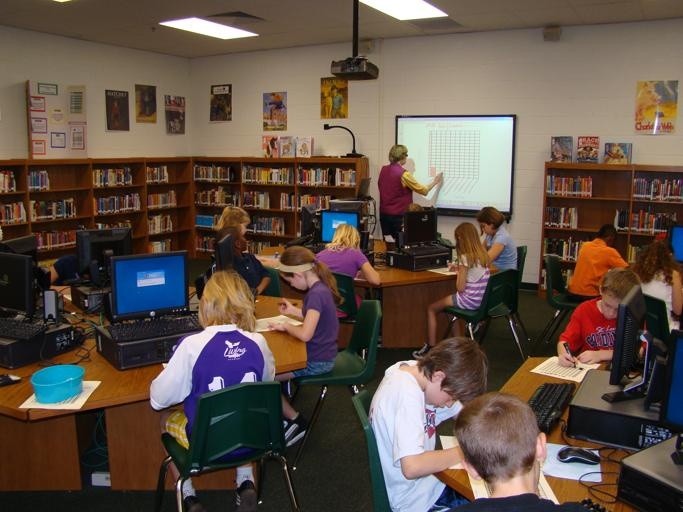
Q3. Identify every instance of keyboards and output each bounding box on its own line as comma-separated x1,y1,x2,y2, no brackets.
108,318,204,343
401,246,449,256
288,237,309,245
0,319,48,341
528,383,576,434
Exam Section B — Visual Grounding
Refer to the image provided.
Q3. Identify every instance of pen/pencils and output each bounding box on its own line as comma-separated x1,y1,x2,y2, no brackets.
278,302,297,306
563,342,577,369
447,259,458,273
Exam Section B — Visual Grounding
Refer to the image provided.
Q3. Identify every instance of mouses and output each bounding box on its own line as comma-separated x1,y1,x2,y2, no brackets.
557,447,600,465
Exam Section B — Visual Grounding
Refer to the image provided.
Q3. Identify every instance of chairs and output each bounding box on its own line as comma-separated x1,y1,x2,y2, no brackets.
351,389,394,512
441,246,580,364
290,300,383,472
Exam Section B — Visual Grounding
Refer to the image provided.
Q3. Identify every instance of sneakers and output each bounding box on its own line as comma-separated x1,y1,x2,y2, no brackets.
282,410,307,448
412,342,435,360
233,481,258,511
182,496,203,512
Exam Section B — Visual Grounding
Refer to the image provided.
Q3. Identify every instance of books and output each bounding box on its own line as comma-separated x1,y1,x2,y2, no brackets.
546,175,683,202
263,136,278,159
614,208,683,263
551,136,572,163
541,209,583,287
604,142,633,165
296,136,311,158
279,136,295,158
577,135,600,164
0,165,356,254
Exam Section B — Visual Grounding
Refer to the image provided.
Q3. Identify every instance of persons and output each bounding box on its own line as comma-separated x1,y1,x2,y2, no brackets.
378,145,443,242
411,206,517,359
557,216,683,368
269,245,340,449
316,223,381,319
149,269,276,512
324,91,332,119
330,84,346,118
50,254,78,285
369,336,606,512
195,227,272,300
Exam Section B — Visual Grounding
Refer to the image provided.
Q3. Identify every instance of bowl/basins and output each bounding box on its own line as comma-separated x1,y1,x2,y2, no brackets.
31,362,86,403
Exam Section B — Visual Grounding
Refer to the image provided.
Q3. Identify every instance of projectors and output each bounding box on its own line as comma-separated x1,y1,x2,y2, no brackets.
330,59,378,80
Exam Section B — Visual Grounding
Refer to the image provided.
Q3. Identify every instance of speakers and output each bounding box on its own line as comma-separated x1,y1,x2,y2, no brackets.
37,267,51,289
43,289,58,324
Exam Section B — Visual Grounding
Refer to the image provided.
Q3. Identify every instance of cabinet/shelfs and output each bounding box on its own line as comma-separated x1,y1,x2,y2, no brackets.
537,161,682,301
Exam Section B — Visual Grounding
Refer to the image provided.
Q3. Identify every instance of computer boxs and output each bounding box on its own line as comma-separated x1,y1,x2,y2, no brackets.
386,251,452,272
617,434,683,512
0,314,77,369
95,325,205,370
568,369,679,451
71,283,112,313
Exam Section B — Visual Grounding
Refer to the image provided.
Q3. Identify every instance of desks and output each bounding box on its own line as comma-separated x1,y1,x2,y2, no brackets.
254,239,466,350
432,356,644,512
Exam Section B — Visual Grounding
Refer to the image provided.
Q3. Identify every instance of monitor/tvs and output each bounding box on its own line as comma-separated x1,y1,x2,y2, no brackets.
0,253,33,313
76,228,132,276
110,250,191,323
301,200,368,242
0,236,36,279
403,211,437,244
601,224,683,431
